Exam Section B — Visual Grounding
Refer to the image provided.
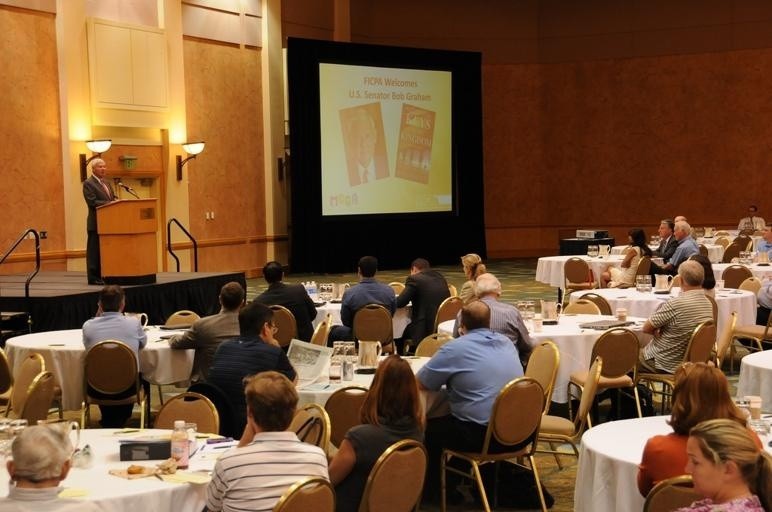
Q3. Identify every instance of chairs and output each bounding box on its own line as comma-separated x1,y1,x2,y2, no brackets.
157,310,206,399
238,280,454,458
356,439,432,512
526,356,602,469
438,375,549,512
433,231,772,412
78,338,152,430
566,326,643,432
270,475,339,512
0,345,66,422
154,392,222,434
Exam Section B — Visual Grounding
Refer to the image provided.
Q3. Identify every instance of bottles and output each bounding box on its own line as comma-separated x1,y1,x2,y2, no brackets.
730,393,763,420
342,356,353,382
329,358,342,383
171,418,190,466
301,281,318,300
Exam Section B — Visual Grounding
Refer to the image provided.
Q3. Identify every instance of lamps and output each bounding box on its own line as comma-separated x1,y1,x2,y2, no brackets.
176,142,205,182
80,140,112,183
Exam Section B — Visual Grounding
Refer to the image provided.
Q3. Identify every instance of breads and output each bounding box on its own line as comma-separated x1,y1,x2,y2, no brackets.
128,464,145,475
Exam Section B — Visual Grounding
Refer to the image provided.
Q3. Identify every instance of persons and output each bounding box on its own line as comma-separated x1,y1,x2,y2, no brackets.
757,221,772,253
416,300,526,504
602,216,699,289
637,362,748,497
738,206,766,229
212,302,300,437
0,424,99,511
687,253,716,300
83,158,116,285
83,284,147,428
170,281,245,385
328,354,428,511
678,418,771,511
327,252,533,354
604,260,713,420
207,371,329,512
737,248,771,353
347,111,386,183
252,260,317,343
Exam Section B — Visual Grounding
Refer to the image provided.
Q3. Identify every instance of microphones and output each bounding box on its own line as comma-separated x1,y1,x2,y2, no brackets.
118,182,134,191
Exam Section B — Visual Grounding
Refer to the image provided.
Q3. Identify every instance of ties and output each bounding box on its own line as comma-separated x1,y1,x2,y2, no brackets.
101,179,112,201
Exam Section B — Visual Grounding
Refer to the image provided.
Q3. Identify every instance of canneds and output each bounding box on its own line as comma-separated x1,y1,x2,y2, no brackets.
343,362,354,381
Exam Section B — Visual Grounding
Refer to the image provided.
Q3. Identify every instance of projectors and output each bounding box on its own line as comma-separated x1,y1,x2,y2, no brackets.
576,230,609,239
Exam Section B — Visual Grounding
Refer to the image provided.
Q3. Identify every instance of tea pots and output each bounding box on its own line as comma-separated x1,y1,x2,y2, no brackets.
124,311,148,331
539,298,562,325
598,245,611,256
333,282,351,300
37,418,81,452
756,249,772,266
653,274,674,294
702,227,716,238
358,340,383,374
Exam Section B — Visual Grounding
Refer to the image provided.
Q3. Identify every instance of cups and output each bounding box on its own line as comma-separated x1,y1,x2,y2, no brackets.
186,422,198,457
739,250,752,269
586,244,598,257
533,318,543,331
1,416,30,457
331,340,356,363
636,274,652,296
616,309,627,321
518,300,536,320
749,418,770,446
713,279,725,294
319,283,333,299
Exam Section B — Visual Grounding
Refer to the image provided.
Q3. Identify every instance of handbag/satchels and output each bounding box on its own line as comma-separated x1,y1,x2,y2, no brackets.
621,383,654,418
